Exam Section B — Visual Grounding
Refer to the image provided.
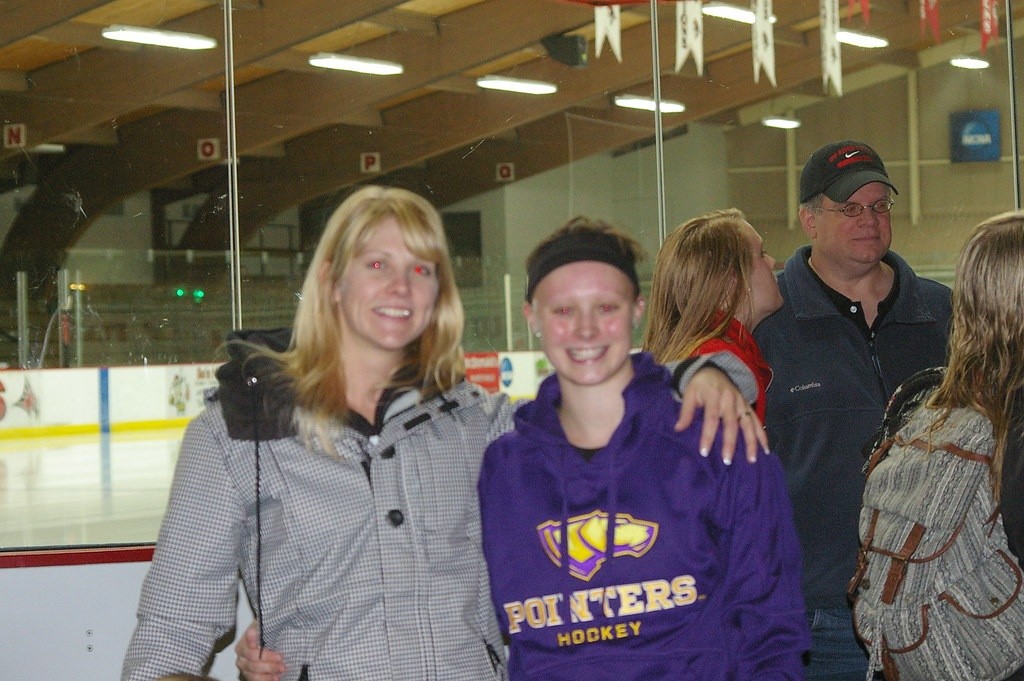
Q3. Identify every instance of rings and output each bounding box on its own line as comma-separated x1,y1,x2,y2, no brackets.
737,411,754,423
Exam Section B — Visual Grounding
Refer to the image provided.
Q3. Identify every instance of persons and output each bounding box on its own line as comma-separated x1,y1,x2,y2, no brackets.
120,185,772,680
480,218,812,679
861,210,1024,681
641,142,963,680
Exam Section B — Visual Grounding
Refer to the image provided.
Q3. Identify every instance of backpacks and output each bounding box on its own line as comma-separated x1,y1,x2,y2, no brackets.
848,365,1024,681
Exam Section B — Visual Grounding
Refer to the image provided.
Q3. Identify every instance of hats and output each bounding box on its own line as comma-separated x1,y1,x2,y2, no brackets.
800,140,900,204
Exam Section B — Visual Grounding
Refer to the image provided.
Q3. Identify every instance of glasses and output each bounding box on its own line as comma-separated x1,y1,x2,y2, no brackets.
809,195,895,217
